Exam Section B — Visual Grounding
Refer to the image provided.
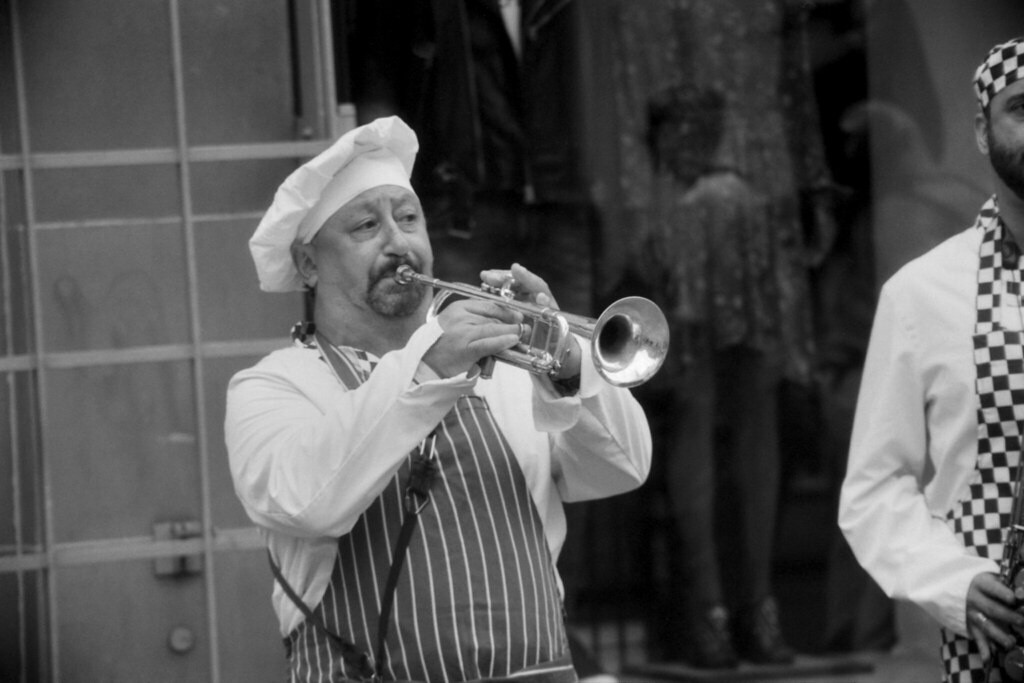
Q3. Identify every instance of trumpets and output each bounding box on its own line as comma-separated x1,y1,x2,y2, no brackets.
393,262,674,392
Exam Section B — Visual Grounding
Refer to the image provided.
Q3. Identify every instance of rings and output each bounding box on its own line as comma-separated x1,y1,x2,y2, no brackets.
974,611,986,627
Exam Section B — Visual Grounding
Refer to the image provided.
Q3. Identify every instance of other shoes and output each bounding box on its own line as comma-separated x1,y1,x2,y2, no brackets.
686,608,736,669
745,607,794,666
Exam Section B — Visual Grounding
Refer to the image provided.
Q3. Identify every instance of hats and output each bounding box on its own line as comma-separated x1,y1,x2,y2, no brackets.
249,116,419,293
973,36,1024,107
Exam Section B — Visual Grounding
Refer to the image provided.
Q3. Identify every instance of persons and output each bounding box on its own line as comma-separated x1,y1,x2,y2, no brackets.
352,1,817,667
225,113,655,683
838,38,1024,683
